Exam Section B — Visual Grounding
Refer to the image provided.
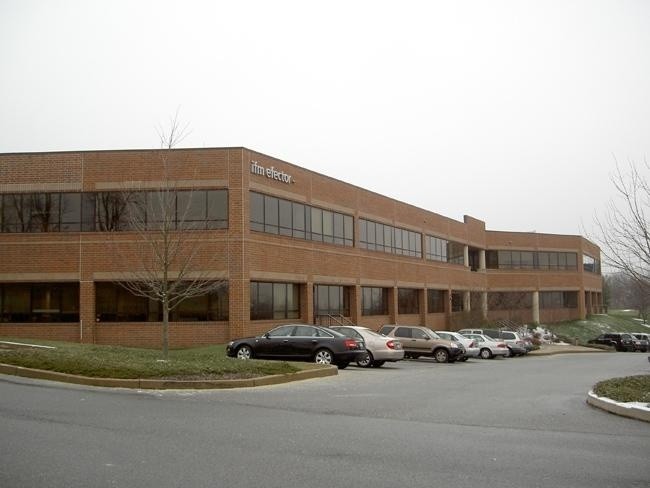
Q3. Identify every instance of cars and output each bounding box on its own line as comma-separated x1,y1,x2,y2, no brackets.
461,333,508,360
315,325,405,367
227,323,366,370
631,334,649,352
421,329,480,361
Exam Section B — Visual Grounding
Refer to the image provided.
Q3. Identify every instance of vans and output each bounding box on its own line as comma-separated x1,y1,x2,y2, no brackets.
456,327,503,342
632,332,650,352
588,332,634,352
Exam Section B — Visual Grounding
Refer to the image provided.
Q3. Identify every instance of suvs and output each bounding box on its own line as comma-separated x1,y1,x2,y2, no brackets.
501,330,527,358
379,323,464,363
